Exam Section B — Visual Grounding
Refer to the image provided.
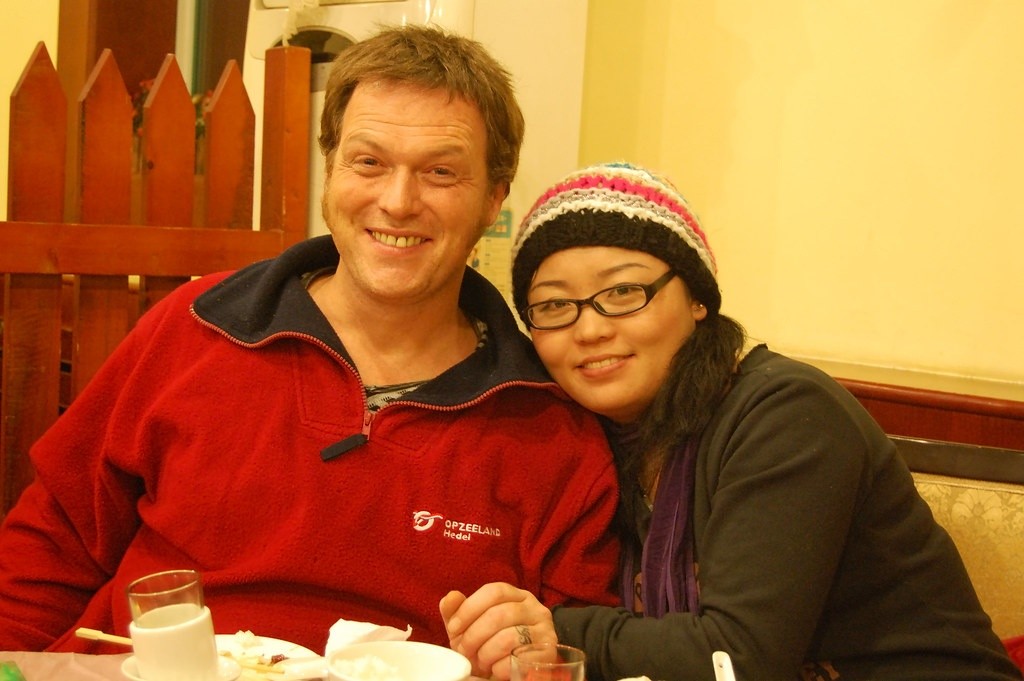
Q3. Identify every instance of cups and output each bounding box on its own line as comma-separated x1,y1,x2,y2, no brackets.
510,643,586,681
325,641,471,681
129,603,223,681
126,570,204,628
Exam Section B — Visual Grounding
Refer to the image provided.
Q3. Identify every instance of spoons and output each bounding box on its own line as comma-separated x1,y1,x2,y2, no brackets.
75,627,241,681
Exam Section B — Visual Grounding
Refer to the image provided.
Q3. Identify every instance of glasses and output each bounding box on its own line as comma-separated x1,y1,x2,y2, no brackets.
519,269,677,330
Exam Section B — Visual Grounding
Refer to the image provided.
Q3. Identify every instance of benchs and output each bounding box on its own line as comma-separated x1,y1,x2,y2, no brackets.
889,432,1023,646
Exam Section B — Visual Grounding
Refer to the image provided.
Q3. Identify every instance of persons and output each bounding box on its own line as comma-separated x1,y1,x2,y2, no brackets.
509,161,1024,681
0,27,620,681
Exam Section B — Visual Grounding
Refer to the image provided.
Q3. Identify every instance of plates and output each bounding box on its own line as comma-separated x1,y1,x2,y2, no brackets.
121,634,323,681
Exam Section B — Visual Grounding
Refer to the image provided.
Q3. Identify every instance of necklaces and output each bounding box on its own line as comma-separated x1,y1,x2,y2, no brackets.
635,455,660,499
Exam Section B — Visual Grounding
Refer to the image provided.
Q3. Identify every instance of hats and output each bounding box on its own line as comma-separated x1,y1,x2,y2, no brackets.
511,163,721,331
